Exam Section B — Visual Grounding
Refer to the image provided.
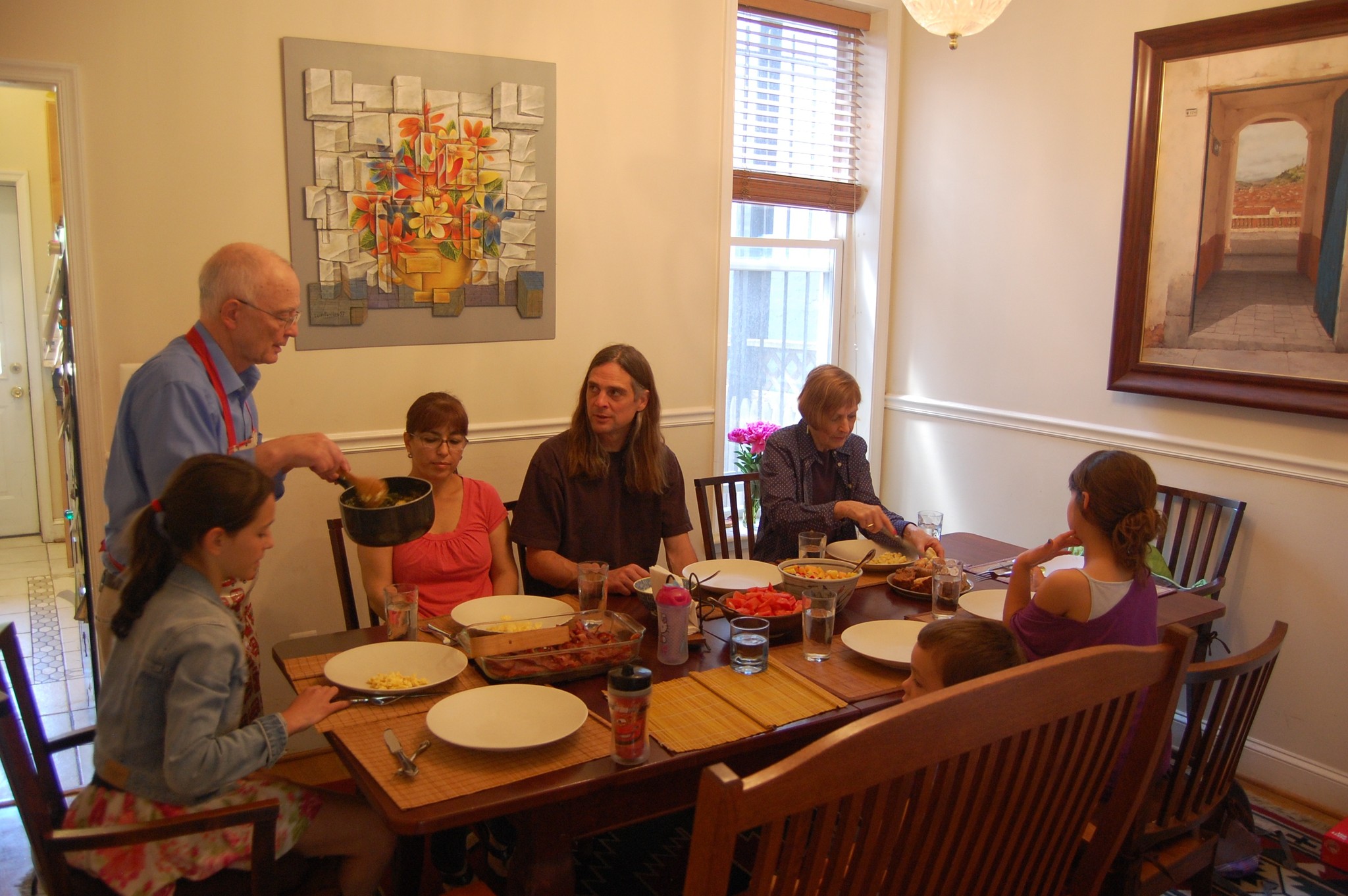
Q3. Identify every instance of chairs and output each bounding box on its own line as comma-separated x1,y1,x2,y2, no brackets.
693,469,767,565
505,616,1292,896
499,497,541,599
1148,481,1249,749
0,616,349,896
324,515,383,635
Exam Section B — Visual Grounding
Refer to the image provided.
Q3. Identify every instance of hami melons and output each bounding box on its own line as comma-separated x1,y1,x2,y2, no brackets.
798,565,846,580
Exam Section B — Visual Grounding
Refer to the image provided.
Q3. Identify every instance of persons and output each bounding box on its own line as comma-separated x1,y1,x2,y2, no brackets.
53,453,397,896
356,391,519,621
748,364,945,564
98,245,350,757
752,619,1026,896
508,342,700,598
1003,449,1172,802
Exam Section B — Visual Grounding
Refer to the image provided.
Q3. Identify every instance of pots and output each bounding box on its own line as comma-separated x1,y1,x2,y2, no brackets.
338,476,435,547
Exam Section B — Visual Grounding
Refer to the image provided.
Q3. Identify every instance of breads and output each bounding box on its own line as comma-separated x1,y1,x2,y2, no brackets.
892,547,970,595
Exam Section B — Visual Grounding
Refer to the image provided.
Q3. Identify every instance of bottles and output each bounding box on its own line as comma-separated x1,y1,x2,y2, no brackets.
656,584,689,665
607,663,654,766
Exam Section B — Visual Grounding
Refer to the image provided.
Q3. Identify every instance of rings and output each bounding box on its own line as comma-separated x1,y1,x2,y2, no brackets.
867,523,874,527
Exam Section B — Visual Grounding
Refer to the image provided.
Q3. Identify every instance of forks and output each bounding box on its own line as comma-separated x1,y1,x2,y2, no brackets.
974,566,1046,583
419,626,459,646
391,741,432,776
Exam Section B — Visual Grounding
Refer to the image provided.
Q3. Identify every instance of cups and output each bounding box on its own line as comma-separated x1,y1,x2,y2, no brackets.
918,510,945,542
382,583,419,642
932,558,964,619
802,589,838,661
798,532,827,559
729,616,769,675
578,561,610,611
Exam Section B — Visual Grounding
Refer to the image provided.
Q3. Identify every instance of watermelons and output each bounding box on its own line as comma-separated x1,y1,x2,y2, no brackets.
724,582,811,616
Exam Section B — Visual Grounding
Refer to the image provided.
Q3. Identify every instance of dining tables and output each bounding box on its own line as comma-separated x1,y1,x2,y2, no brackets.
268,526,1229,896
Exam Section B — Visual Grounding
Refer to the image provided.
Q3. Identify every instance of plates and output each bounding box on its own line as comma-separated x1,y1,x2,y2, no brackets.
682,558,783,593
841,619,930,670
426,683,588,752
826,539,918,572
451,595,575,636
958,590,1036,624
887,572,974,600
324,641,468,695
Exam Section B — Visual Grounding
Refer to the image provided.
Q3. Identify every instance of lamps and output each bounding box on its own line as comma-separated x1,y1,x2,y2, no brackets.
899,0,1015,51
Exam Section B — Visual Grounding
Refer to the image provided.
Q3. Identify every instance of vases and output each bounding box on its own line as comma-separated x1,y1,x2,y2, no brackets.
742,478,766,530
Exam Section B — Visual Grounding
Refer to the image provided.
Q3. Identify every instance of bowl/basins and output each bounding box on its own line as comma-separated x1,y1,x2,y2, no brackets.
456,610,647,682
633,576,697,620
715,557,863,639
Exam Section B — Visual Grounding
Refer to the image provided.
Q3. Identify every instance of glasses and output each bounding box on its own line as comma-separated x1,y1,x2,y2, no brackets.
410,430,469,450
218,298,301,332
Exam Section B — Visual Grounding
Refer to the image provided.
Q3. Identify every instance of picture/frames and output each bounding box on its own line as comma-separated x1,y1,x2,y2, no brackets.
1102,0,1348,428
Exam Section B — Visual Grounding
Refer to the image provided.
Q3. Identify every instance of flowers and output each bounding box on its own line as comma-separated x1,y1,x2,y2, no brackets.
726,419,783,523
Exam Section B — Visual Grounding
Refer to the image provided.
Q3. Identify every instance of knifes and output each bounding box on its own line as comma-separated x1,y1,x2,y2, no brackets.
384,728,420,776
